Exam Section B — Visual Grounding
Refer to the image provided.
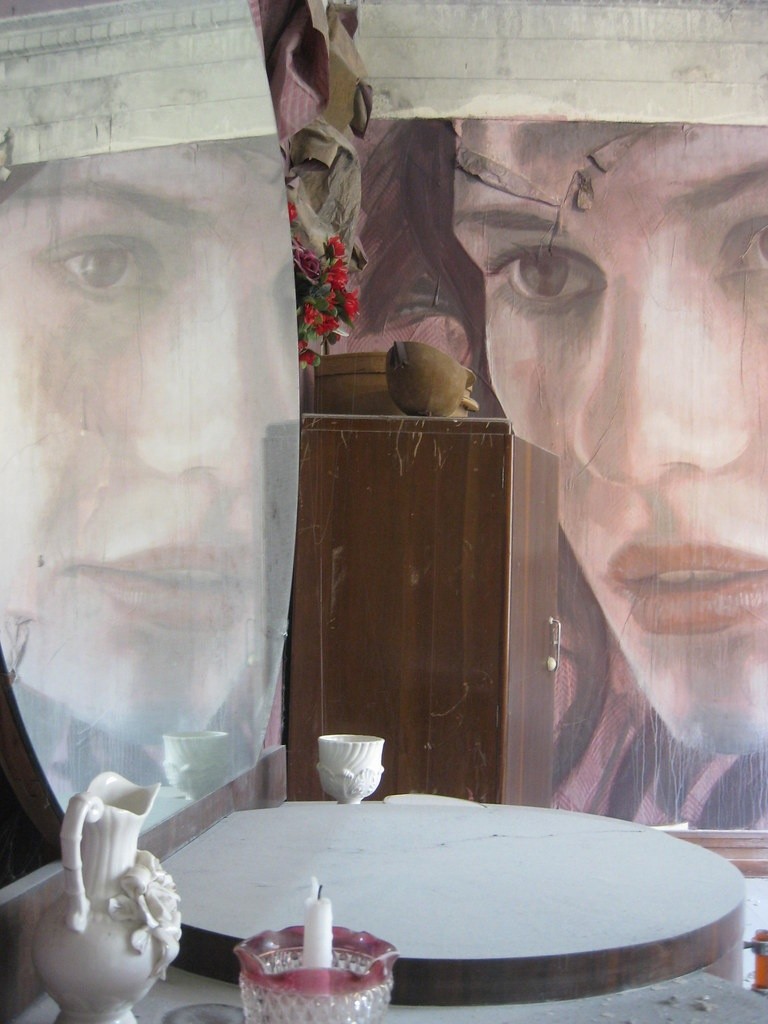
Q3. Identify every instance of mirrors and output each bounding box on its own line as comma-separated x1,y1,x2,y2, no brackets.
1,0,301,862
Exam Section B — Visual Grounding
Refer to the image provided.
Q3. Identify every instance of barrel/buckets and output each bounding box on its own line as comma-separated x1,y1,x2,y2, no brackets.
314,351,477,419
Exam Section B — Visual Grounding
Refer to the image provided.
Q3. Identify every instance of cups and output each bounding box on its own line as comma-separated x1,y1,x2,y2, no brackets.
316,732,386,803
162,730,231,801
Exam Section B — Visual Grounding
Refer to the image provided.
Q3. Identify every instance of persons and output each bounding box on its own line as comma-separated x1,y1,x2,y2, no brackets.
1,136,295,794
442,118,767,776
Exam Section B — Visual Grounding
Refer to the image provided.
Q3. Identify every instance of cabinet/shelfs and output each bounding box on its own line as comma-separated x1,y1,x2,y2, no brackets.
285,415,561,810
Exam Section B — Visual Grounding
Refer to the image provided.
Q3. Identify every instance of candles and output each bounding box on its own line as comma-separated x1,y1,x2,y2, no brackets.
301,880,335,975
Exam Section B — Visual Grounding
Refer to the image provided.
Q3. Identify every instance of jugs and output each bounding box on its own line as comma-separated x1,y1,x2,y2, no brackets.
31,770,181,1023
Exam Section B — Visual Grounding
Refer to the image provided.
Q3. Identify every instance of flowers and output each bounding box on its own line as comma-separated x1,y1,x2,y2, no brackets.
290,201,361,369
